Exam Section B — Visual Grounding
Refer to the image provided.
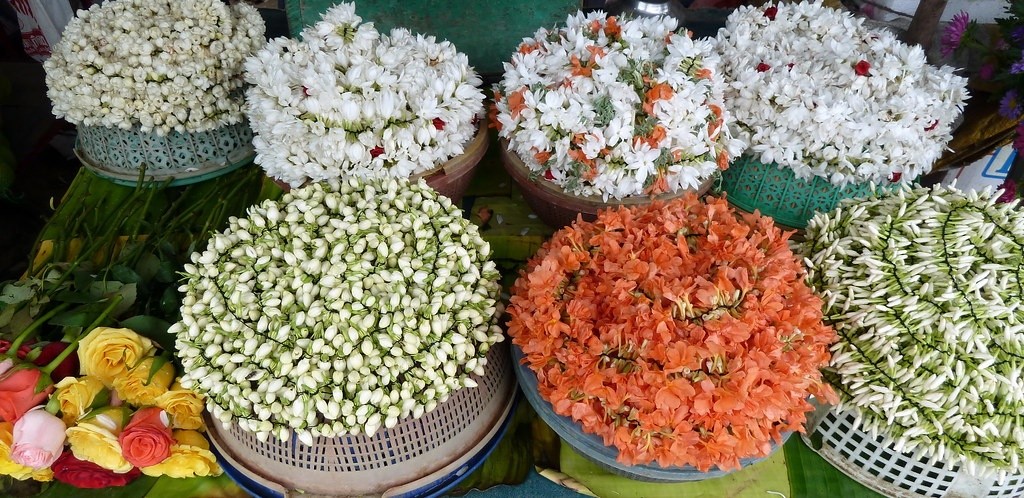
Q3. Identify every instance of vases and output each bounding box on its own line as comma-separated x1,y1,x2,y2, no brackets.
199,334,518,498
497,129,718,231
268,113,491,206
71,119,255,191
509,333,793,483
707,153,920,244
801,387,1024,498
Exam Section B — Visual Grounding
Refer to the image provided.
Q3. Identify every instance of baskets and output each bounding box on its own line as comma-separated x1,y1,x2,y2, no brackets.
271,117,490,205
797,394,1023,498
511,345,811,483
501,138,715,230
712,152,906,243
72,83,257,188
201,318,521,498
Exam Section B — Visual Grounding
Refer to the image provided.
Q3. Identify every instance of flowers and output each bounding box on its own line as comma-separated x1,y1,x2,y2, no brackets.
0,0,1024,498
